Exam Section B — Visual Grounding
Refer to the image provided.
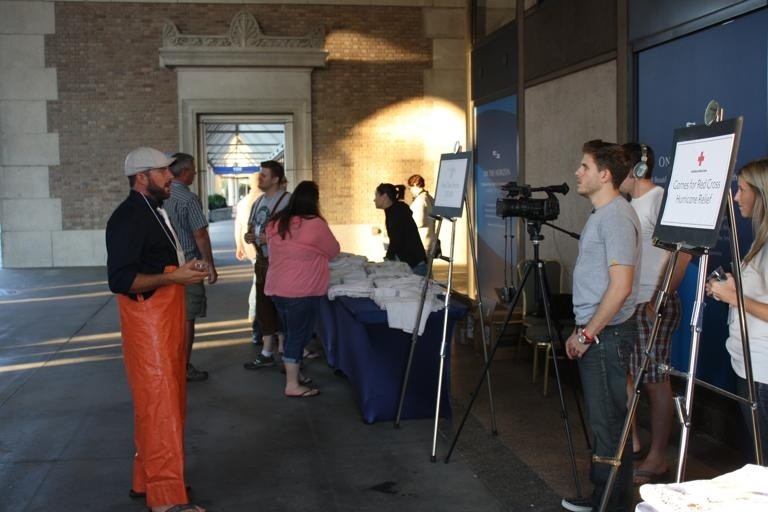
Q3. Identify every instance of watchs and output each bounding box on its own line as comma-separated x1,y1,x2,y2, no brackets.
577,327,600,346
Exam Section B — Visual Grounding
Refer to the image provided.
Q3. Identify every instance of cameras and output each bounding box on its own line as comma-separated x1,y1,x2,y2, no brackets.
705,266,728,282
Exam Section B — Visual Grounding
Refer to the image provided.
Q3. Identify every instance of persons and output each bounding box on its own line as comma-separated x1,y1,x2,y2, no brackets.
104,147,209,511
374,184,432,279
705,159,768,464
233,161,342,398
560,140,642,511
621,143,692,485
407,175,437,262
163,151,219,379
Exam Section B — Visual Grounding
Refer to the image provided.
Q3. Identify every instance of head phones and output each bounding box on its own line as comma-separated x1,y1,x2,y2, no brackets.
634,141,650,182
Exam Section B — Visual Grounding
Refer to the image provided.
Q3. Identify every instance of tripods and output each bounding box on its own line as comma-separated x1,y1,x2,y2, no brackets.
445,240,592,499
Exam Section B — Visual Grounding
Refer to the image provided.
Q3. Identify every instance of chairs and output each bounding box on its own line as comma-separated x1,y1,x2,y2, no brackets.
516,257,565,371
525,326,571,400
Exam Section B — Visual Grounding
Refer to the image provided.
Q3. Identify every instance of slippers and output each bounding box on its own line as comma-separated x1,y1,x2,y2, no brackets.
148,503,206,512
632,462,670,484
130,480,190,498
632,451,640,458
242,327,320,397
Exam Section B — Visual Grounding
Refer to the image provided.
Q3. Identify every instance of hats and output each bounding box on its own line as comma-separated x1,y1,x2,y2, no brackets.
124,147,178,177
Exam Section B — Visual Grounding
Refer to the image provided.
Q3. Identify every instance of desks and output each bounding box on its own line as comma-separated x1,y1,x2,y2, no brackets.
313,258,471,425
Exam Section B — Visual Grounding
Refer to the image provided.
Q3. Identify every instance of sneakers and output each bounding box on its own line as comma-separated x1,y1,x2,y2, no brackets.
562,497,596,511
186,365,209,381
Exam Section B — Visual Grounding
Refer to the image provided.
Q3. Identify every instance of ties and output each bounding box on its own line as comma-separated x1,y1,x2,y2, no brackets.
157,207,186,268
